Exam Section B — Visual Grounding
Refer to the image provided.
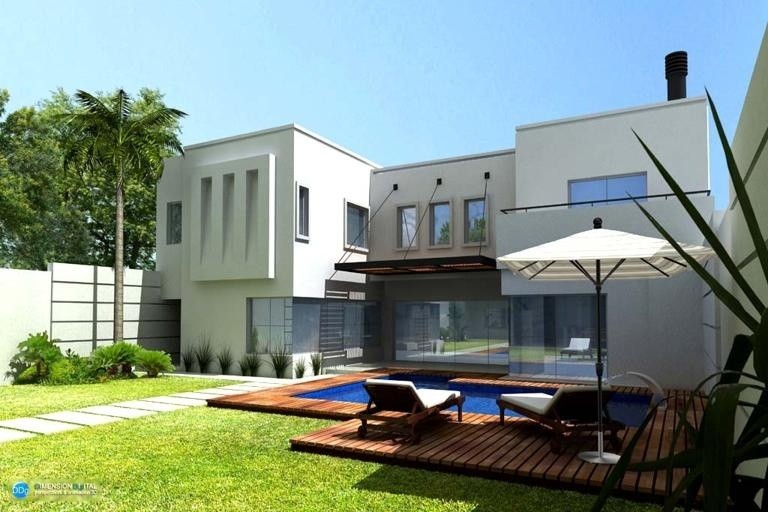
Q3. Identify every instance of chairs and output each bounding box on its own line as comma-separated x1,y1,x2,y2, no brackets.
356,379,465,446
495,385,623,456
560,337,593,360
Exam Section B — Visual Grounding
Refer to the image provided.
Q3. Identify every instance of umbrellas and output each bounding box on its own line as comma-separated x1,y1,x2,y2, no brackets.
496,218,716,458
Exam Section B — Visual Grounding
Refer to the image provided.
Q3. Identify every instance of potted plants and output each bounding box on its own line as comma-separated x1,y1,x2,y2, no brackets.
135,348,177,378
